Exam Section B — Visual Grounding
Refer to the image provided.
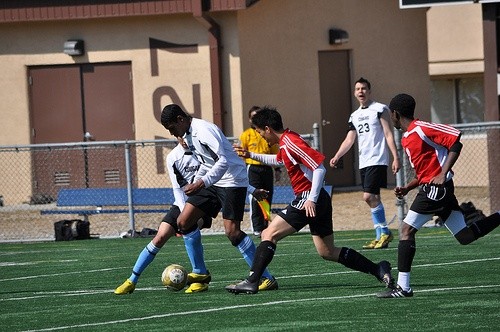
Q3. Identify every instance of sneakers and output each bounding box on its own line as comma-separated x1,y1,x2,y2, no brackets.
223,279,259,295
376,232,394,248
375,284,414,299
115,278,135,295
186,270,212,284
258,279,280,291
185,283,209,293
377,260,395,288
362,239,380,249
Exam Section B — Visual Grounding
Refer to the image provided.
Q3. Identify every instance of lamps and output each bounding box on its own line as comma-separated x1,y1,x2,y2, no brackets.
329,29,349,46
64,40,85,57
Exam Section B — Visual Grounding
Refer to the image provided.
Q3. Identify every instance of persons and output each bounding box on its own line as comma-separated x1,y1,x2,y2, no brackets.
114,135,212,295
377,94,500,298
237,104,281,236
160,104,278,291
225,107,395,295
329,77,402,248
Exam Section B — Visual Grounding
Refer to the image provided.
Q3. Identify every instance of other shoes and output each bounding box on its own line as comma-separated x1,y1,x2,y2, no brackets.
253,231,263,238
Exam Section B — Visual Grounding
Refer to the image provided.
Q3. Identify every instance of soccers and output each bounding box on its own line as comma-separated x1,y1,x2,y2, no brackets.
162,264,187,291
